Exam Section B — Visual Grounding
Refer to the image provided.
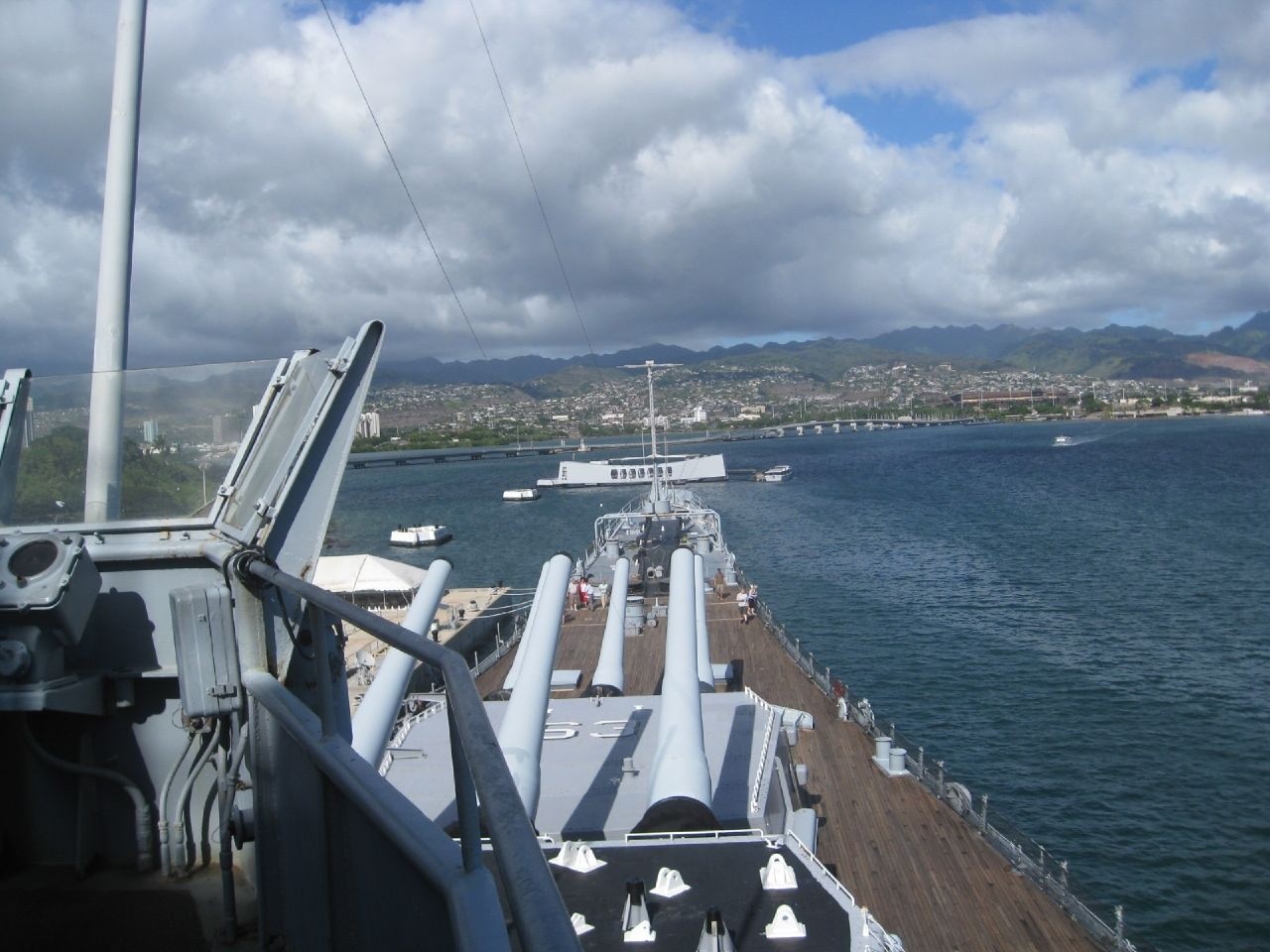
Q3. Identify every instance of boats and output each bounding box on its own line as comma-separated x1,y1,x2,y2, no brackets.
1053,435,1073,446
764,464,794,483
502,487,540,502
388,522,453,548
537,436,731,488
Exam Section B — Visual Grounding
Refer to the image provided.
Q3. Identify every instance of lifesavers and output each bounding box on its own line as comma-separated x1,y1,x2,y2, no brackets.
833,680,844,696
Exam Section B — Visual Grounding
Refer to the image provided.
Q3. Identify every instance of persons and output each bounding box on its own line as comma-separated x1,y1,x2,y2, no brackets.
599,581,609,609
714,568,725,601
566,577,595,611
736,583,757,624
